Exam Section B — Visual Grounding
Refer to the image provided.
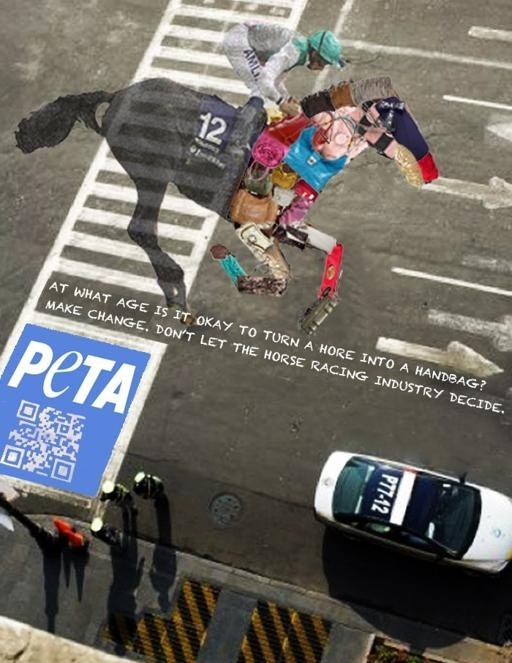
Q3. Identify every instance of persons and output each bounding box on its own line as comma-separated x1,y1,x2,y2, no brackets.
90,519,122,551
100,481,137,518
224,22,340,155
133,472,167,505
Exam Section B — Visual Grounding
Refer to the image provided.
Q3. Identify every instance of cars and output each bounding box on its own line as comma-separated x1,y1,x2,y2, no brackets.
313,448,511,574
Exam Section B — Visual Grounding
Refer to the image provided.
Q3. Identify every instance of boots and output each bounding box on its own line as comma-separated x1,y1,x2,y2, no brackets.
223,102,260,161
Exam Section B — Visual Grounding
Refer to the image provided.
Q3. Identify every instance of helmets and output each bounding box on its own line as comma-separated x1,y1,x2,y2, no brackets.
307,29,341,67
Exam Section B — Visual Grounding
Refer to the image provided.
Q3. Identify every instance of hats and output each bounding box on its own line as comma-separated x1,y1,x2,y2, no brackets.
134,470,145,484
102,479,115,495
90,518,104,532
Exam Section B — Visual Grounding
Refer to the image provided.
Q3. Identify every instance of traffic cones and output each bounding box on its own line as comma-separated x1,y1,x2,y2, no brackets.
62,528,88,552
53,517,76,540
